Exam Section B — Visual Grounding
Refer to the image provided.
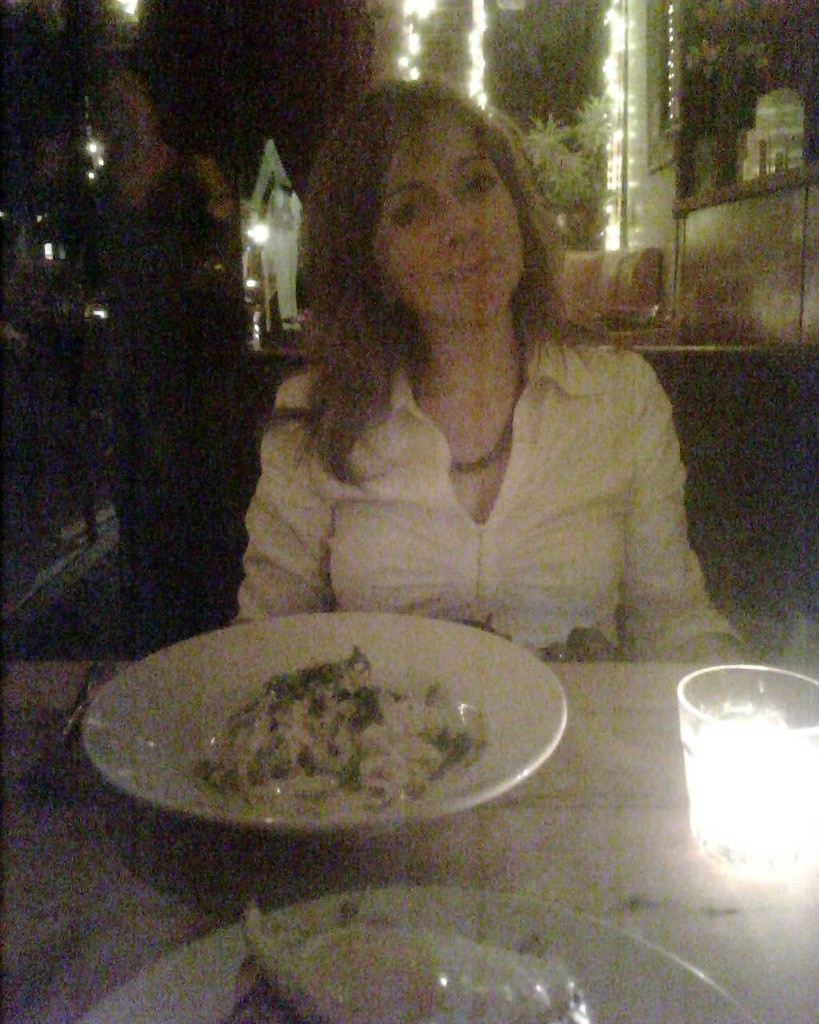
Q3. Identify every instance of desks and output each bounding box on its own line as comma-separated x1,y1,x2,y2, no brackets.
198,345,819,622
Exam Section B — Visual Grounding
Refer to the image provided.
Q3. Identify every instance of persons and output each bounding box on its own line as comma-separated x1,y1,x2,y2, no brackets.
85,8,240,573
236,79,745,668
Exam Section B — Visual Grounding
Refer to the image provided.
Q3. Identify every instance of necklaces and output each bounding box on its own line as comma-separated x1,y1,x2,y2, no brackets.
449,369,522,472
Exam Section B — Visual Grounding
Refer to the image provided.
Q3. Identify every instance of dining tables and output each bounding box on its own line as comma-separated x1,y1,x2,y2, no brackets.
0,660,819,1024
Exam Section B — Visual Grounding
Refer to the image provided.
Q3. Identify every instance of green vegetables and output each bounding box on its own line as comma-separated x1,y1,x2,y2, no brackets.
196,643,475,787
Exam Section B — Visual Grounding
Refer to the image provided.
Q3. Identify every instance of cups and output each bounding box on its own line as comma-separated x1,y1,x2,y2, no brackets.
676,665,819,880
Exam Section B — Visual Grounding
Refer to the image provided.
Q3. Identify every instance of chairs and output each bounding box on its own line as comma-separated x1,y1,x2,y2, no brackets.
107,374,217,617
2,336,108,559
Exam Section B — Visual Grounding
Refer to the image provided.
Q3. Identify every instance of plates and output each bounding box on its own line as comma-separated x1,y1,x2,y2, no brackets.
79,613,568,834
73,887,756,1024
